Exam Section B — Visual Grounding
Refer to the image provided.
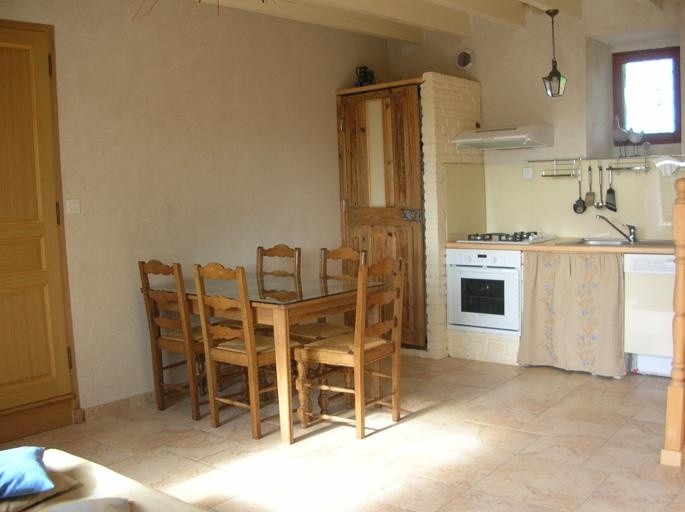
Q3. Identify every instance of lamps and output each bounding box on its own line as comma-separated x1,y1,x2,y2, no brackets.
542,9,566,98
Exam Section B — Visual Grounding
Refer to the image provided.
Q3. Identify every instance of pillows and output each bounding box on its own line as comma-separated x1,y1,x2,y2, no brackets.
0,445,57,501
0,466,78,512
39,497,130,512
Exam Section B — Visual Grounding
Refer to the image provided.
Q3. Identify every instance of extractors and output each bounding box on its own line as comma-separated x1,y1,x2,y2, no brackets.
449,122,552,152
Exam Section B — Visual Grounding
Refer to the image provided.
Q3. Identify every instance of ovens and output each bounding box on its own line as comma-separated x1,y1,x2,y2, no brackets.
445,247,525,337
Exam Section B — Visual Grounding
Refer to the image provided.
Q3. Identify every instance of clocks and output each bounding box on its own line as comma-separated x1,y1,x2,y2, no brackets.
454,48,474,70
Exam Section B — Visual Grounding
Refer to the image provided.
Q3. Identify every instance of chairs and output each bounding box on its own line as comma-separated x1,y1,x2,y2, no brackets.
193,262,302,439
217,243,301,400
264,246,368,402
137,260,248,421
294,256,405,439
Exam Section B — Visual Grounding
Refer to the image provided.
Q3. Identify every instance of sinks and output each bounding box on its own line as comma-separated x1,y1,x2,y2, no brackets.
582,239,622,245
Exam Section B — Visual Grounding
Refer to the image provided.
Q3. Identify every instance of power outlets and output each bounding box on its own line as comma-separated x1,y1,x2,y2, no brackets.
66,199,80,215
522,168,534,181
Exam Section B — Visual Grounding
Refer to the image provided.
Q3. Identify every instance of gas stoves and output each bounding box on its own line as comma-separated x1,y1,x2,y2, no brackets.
465,229,537,244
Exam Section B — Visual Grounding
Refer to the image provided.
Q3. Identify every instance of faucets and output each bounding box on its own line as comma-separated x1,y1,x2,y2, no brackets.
596,215,636,244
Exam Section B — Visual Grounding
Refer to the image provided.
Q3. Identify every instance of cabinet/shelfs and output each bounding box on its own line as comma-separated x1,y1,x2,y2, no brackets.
623,254,677,379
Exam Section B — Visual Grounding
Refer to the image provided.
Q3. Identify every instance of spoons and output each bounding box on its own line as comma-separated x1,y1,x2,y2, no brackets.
593,165,606,209
571,169,587,216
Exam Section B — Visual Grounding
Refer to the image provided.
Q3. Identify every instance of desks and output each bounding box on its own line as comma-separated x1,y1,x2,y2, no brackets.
140,272,387,446
